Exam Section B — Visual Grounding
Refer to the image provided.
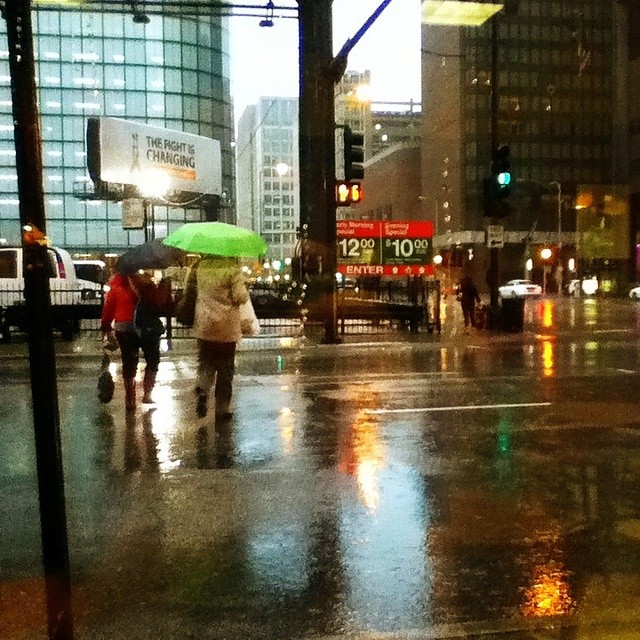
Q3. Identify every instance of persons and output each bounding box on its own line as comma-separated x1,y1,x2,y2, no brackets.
99,272,163,407
458,277,482,328
186,258,246,422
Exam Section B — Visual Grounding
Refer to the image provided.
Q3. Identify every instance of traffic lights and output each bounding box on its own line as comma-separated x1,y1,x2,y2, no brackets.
343,124,365,181
337,179,350,206
350,181,361,203
491,142,512,198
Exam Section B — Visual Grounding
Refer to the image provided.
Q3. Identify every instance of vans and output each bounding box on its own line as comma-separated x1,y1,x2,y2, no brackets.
0,244,81,336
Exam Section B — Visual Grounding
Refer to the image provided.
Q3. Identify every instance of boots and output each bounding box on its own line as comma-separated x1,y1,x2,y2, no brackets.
142,373,155,404
123,378,138,411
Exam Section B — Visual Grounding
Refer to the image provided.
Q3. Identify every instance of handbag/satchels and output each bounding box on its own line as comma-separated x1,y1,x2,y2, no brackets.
134,297,166,340
177,258,200,326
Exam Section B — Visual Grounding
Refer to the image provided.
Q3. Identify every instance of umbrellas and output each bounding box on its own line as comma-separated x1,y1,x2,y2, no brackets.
161,222,269,257
115,240,187,269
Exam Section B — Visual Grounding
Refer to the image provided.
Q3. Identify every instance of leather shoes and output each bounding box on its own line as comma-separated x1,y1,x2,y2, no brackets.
197,392,208,419
215,411,233,425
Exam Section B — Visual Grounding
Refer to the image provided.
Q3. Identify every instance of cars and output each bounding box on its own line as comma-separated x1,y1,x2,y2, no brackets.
498,279,542,300
629,287,640,301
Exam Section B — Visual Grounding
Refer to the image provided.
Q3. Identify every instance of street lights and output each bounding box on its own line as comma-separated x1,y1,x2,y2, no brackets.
541,248,552,296
276,163,289,273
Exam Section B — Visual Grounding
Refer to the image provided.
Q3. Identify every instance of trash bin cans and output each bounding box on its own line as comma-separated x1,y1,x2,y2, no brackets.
502,298,524,333
